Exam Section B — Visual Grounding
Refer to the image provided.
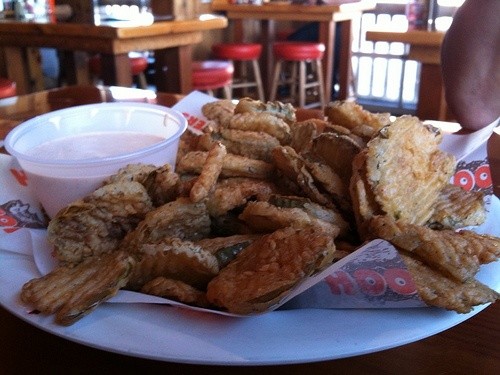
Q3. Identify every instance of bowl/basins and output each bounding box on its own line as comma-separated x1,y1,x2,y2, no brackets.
4,102,189,221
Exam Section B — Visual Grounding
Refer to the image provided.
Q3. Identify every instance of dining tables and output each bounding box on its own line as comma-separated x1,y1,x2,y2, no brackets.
1,84,500,375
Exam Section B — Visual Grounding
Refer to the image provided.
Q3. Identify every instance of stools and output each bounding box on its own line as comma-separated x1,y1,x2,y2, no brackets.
88,43,326,110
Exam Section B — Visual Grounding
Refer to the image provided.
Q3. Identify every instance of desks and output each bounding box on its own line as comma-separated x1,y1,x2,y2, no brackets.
211,0,378,102
365,31,452,124
0,17,229,95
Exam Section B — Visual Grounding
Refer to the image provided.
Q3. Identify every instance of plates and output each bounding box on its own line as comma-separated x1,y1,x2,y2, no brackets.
1,193,500,366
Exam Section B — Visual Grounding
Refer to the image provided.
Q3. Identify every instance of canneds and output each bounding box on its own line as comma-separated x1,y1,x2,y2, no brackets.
408,0,437,31
15,0,56,23
